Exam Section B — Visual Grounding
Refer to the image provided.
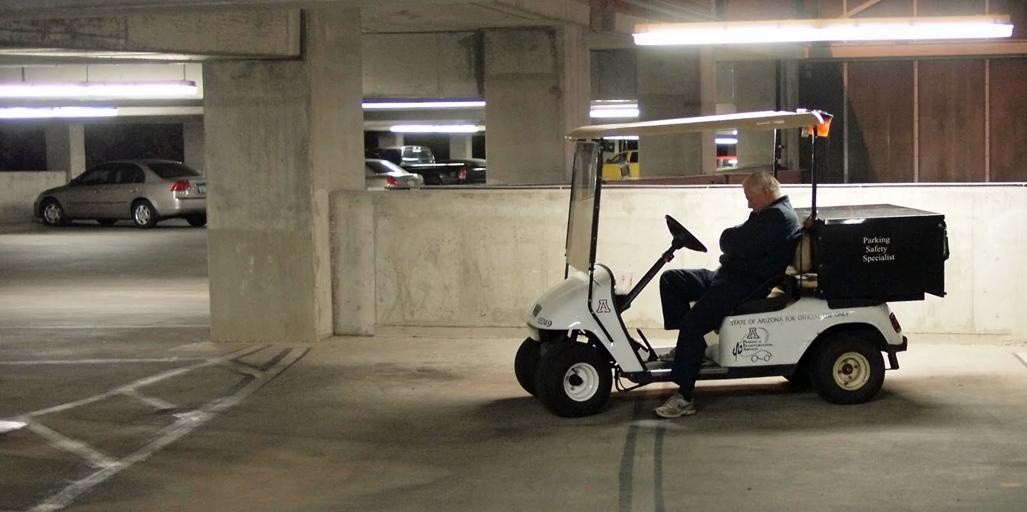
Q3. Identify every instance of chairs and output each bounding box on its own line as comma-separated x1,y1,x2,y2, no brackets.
729,233,800,314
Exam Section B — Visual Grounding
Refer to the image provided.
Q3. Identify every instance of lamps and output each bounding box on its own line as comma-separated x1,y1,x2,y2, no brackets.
391,122,480,134
631,13,1013,47
0,64,199,119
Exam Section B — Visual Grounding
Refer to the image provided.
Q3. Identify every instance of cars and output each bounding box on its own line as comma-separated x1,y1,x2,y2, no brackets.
34,158,206,228
365,159,424,191
445,158,487,182
716,154,738,169
602,149,640,184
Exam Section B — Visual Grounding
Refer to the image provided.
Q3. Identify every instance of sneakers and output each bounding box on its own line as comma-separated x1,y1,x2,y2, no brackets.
652,390,697,419
658,345,677,362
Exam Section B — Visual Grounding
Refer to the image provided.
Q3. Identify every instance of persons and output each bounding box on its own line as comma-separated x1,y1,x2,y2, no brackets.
653,169,802,419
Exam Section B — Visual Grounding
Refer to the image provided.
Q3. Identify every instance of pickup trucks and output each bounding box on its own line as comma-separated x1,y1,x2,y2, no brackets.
377,145,475,185
